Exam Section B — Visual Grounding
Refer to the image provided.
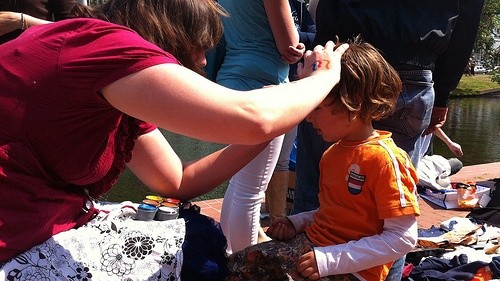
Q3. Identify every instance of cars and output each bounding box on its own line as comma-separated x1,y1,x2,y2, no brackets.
473,66,487,73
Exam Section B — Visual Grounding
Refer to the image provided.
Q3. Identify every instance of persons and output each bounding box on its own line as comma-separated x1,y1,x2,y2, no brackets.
0,0,349,281
260,0,316,227
311,0,486,170
217,33,421,281
215,0,306,256
411,128,464,191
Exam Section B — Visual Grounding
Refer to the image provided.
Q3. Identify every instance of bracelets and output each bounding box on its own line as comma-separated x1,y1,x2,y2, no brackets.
21,13,28,29
